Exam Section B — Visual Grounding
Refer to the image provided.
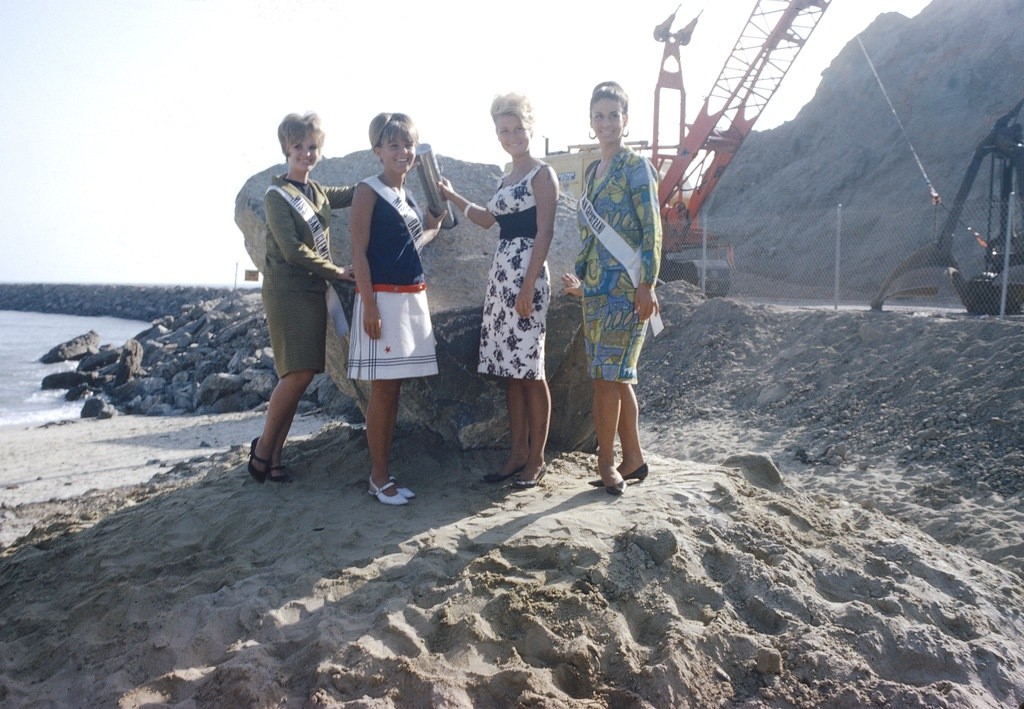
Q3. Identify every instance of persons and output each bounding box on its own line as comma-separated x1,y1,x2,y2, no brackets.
248,112,421,484
561,82,664,497
437,95,559,489
347,112,449,507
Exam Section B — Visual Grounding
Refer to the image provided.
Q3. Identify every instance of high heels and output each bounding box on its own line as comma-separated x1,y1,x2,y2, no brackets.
513,461,546,488
483,460,527,482
588,462,648,487
606,480,627,495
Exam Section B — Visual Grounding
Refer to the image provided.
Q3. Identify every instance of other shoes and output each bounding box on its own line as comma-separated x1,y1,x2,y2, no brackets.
368,475,408,505
269,462,289,483
247,437,271,484
389,476,416,498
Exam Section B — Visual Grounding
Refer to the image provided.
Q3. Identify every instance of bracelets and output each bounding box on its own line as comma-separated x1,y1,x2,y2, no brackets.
463,203,476,218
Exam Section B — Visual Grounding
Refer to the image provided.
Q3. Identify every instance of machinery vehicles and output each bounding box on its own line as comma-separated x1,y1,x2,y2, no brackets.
870,98,1024,315
498,0,836,300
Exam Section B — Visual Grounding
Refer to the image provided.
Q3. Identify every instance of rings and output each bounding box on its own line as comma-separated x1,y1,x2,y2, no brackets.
350,265,352,267
